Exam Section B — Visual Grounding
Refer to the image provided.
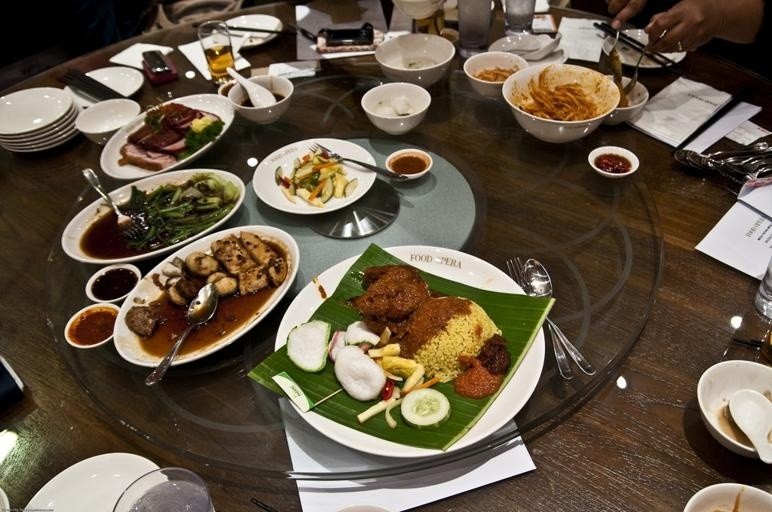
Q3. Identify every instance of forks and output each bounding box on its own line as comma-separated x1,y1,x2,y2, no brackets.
309,141,406,185
504,255,595,381
82,167,143,239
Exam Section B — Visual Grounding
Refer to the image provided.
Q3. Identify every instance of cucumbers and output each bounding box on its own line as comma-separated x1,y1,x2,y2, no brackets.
400,387,452,430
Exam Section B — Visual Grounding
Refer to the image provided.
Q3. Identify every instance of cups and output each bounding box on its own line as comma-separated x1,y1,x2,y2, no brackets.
199,21,239,87
114,467,212,512
755,253,771,319
457,1,491,57
506,1,536,32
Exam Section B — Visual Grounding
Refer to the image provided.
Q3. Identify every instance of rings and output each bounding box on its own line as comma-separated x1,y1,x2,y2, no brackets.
677,40,683,53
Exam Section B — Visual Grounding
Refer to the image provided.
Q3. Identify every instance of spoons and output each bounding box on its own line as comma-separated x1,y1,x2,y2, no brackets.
520,252,573,385
145,283,220,387
225,66,276,108
726,385,771,465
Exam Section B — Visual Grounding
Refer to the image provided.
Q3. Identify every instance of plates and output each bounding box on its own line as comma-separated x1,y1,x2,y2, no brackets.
387,28,460,44
75,66,145,101
275,245,547,460
62,303,121,349
222,15,282,50
100,92,236,180
23,447,164,511
113,226,300,368
1,87,82,154
59,167,246,264
601,29,688,68
587,145,640,181
488,34,569,66
386,148,433,179
84,262,142,304
251,138,377,214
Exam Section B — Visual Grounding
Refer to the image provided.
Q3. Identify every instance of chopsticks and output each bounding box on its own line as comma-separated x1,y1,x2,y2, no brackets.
192,21,286,37
54,66,128,99
592,22,680,68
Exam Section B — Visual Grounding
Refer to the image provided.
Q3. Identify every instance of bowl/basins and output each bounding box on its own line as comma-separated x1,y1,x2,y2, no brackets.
503,62,621,144
684,482,772,512
603,73,649,125
696,360,771,461
230,75,296,124
76,99,141,144
464,51,530,101
375,32,456,87
360,82,433,138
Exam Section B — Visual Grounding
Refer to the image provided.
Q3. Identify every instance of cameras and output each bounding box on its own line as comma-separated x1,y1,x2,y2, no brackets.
314,28,373,46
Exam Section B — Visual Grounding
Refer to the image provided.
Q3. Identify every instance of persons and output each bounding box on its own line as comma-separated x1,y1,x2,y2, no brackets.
0,0,243,92
607,0,771,81
